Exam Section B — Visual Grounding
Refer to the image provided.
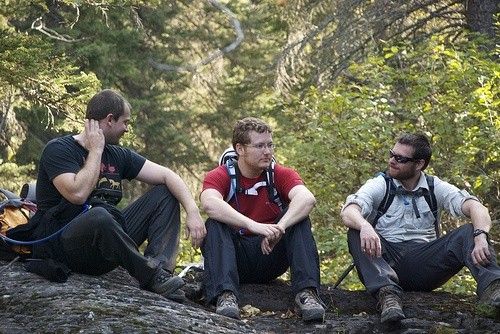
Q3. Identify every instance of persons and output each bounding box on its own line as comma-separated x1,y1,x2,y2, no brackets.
201,117,326,321
33,90,206,300
341,133,500,323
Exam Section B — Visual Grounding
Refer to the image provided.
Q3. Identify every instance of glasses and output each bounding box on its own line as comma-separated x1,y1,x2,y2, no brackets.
241,143,275,150
389,149,423,164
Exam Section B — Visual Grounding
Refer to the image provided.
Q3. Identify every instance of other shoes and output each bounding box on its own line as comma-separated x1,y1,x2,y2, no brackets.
140,269,186,302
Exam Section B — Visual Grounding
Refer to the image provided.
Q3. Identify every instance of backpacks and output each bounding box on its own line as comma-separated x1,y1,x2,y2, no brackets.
0,189,33,258
219,147,285,212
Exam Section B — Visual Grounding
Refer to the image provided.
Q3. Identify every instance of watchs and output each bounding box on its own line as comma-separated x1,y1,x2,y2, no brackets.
473,229,489,238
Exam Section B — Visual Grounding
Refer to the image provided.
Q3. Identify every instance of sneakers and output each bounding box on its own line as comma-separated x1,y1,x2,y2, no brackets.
377,295,405,322
480,283,500,306
294,288,327,321
216,289,241,320
